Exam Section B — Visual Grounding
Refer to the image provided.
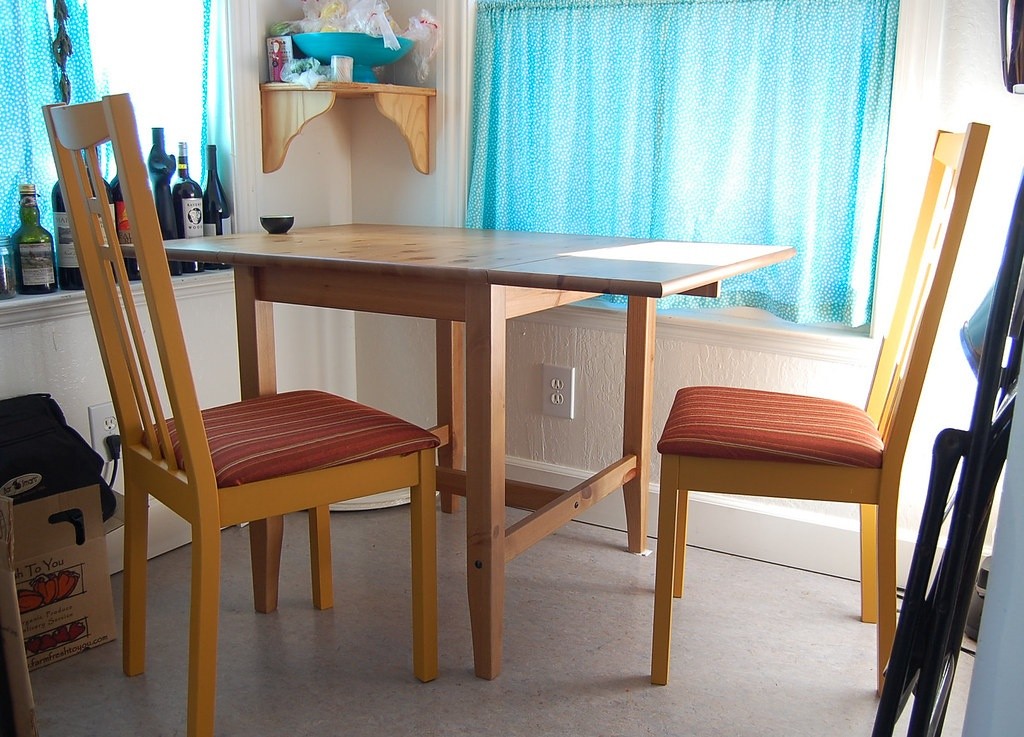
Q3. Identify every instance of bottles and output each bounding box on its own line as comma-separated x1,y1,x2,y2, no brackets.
83,146,118,284
202,144,233,270
12,184,59,295
172,142,204,273
52,179,84,290
109,166,141,281
0,235,16,299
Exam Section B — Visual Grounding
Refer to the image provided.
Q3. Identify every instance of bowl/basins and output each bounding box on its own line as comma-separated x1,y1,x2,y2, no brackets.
291,32,414,83
260,215,294,234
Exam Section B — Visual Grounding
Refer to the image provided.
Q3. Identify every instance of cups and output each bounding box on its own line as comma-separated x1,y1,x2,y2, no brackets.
330,55,354,82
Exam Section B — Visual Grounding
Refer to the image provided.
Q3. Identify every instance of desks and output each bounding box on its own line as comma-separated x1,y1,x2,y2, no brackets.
111,220,797,681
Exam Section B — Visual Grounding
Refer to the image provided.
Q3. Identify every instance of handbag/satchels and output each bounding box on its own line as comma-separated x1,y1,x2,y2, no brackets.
0,393,116,544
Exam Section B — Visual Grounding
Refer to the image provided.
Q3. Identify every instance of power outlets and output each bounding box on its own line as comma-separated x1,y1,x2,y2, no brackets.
543,363,575,419
87,398,124,465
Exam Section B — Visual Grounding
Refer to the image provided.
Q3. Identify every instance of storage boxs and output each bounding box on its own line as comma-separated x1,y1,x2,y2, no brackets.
12,483,118,673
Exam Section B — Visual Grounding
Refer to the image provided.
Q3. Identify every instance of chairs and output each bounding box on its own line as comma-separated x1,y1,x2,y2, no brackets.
651,120,993,684
41,92,440,737
